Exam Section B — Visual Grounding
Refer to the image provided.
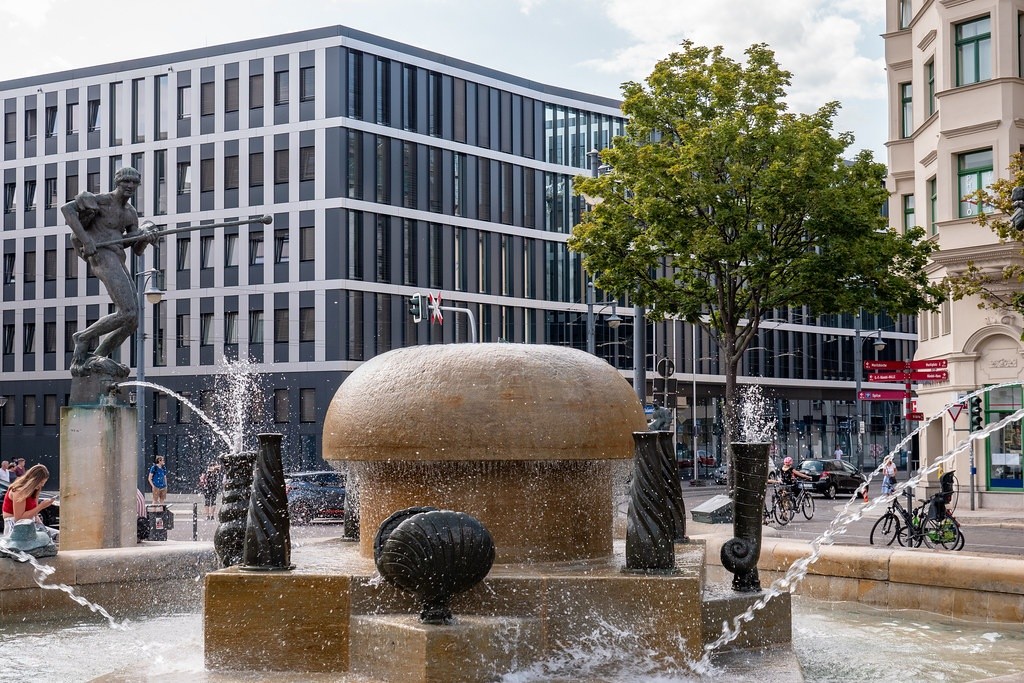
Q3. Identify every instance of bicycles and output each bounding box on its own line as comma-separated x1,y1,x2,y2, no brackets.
869,481,961,552
896,506,966,552
772,477,814,526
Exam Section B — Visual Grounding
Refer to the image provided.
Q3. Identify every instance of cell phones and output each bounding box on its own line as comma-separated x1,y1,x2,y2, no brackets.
49,495,58,500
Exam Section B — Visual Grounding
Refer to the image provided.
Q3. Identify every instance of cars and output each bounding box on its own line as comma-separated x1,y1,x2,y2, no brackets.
795,458,869,499
713,456,777,485
284,471,349,526
0,478,60,528
678,450,718,469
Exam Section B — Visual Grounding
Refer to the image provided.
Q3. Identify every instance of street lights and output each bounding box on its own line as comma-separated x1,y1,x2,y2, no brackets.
855,328,888,473
135,268,166,498
586,300,623,354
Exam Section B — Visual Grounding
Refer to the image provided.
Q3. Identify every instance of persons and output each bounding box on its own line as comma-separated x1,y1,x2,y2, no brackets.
202,460,220,521
136,488,147,543
801,444,814,460
762,457,812,525
834,444,843,459
2,464,56,536
148,456,167,505
882,455,897,496
61,166,159,365
0,458,25,483
1012,427,1022,450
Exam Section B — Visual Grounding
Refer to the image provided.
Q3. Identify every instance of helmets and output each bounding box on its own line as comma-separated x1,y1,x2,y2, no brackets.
784,456,793,465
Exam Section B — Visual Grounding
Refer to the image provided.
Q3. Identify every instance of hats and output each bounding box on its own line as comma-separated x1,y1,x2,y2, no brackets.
17,457,25,462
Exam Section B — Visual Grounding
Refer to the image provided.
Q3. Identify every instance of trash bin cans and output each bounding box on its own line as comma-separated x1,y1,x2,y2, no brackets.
146,505,174,541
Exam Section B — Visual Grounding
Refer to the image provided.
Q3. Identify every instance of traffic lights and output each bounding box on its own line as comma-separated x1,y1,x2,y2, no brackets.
969,396,983,433
408,293,422,323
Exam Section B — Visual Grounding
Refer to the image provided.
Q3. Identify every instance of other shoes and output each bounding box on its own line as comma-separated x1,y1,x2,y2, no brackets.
206,515,215,520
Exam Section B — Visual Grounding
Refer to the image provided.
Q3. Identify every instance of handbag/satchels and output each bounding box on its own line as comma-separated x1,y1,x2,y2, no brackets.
889,476,897,484
